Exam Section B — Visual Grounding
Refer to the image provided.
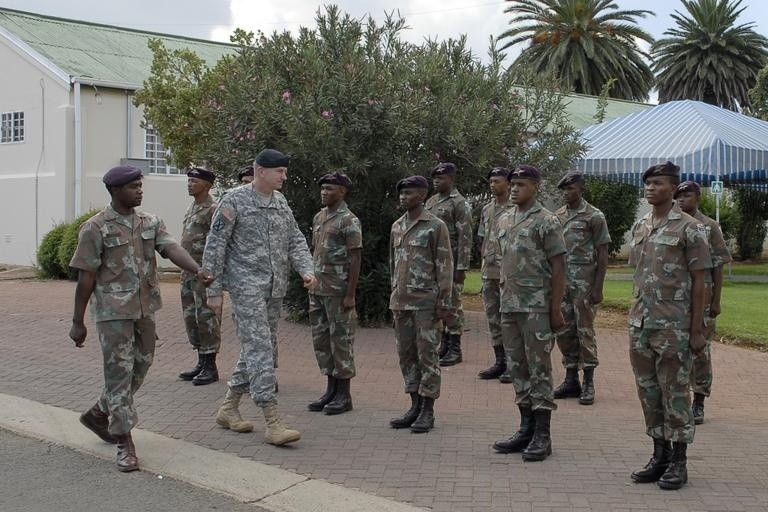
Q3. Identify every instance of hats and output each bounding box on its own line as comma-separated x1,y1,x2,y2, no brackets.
238,166,255,181
487,167,511,181
395,174,429,192
642,160,682,184
255,149,292,169
187,167,216,186
557,169,585,191
429,162,458,178
506,164,542,184
317,171,354,191
672,180,701,201
101,165,143,188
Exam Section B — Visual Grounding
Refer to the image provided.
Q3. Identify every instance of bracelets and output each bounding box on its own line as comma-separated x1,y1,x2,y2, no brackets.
196,267,203,275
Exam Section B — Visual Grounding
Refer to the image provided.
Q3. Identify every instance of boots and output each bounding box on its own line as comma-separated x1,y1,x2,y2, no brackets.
406,396,435,434
436,330,515,385
114,430,140,472
553,366,583,399
177,352,206,380
215,387,255,433
190,352,220,386
491,403,536,453
387,392,424,430
78,399,117,443
690,391,706,424
322,378,353,415
655,440,689,491
577,367,596,406
630,437,674,484
306,375,338,411
261,404,301,446
521,408,554,462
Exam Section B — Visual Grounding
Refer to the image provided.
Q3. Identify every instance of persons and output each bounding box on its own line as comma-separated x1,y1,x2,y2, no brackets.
628,161,712,489
201,149,318,446
389,175,454,432
68,165,215,471
491,165,566,461
477,168,516,383
424,163,473,366
552,171,612,406
238,165,283,393
307,174,363,414
178,168,223,386
674,180,733,423
